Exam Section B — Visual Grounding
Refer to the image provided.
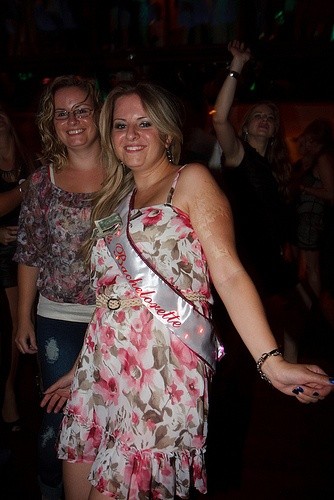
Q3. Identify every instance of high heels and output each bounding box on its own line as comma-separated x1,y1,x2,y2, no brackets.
2,403,23,436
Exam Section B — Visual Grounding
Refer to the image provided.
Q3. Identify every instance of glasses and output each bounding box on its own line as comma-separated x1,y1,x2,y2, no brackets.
51,108,95,120
